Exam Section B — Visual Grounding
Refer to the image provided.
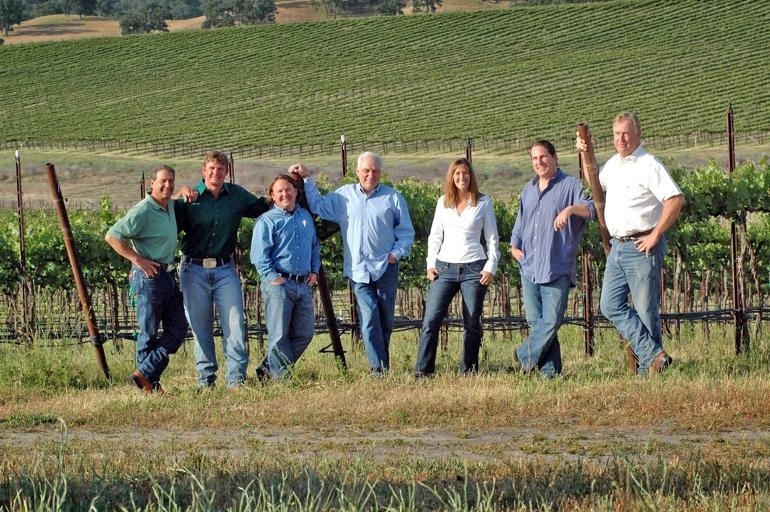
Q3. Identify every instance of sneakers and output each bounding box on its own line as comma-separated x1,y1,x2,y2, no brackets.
651,351,672,372
132,370,165,395
513,350,525,376
256,368,266,387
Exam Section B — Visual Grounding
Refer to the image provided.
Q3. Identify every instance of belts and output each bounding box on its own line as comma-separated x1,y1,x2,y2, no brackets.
282,272,306,282
616,229,651,242
161,264,176,273
186,256,231,269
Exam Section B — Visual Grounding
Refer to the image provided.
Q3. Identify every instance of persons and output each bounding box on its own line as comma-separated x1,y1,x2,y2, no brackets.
104,163,200,393
415,159,501,378
576,109,685,375
512,137,597,379
287,151,416,375
249,172,323,382
174,152,273,389
